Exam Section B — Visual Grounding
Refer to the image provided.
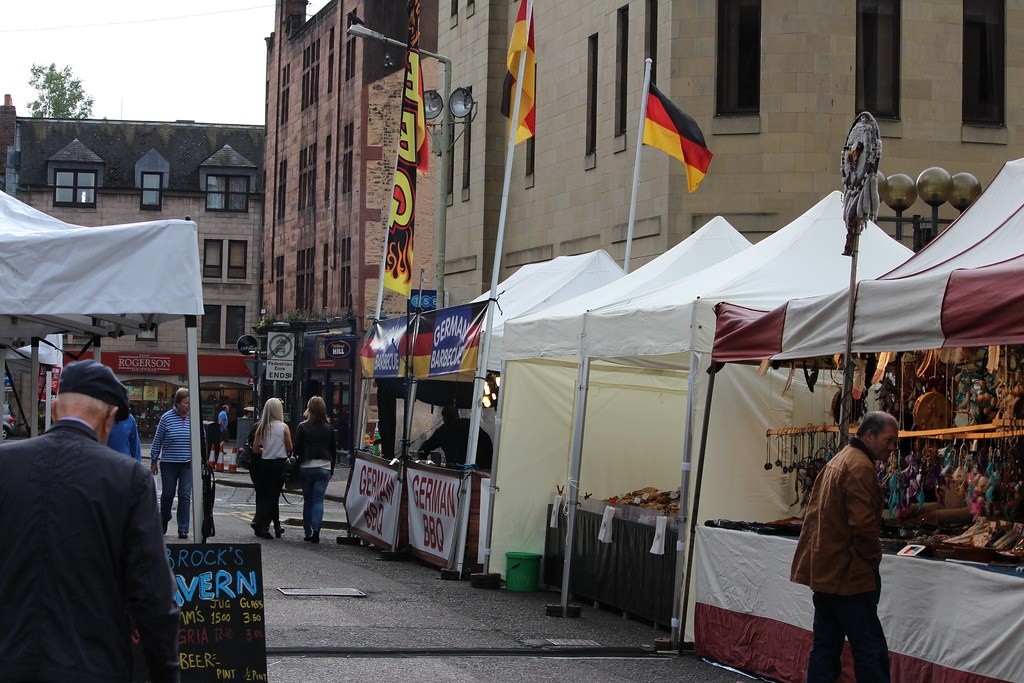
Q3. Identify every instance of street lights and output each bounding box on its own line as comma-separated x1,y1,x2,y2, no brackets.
345,23,455,309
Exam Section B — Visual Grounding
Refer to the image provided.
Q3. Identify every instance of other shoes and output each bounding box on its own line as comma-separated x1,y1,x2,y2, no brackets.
304,535,313,540
163,516,171,534
259,528,273,539
275,526,284,538
179,530,188,539
313,531,321,544
250,522,261,537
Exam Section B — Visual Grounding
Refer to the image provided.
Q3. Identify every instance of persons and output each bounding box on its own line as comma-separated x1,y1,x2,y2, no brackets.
214,396,334,543
150,387,191,540
788,410,897,683
0,361,180,683
107,409,142,462
415,400,493,474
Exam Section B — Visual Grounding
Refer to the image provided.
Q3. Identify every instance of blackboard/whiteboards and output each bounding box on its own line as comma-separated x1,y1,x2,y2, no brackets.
164,543,268,683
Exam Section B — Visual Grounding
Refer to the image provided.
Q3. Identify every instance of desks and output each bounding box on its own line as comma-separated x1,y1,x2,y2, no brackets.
348,451,489,567
542,494,678,628
691,525,1024,683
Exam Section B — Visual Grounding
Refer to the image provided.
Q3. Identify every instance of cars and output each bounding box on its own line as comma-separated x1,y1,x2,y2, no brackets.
2,403,16,440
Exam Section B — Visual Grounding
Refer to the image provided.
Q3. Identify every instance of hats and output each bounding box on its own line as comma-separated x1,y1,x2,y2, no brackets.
58,360,131,424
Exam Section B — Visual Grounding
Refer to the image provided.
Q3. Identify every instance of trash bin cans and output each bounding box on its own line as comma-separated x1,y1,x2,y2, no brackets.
203,420,221,463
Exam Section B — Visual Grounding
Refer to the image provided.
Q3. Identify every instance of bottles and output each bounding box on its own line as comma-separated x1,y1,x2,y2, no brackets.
373,432,379,455
364,432,370,452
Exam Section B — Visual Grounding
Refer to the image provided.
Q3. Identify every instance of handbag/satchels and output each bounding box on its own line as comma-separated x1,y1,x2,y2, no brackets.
284,450,302,490
239,438,253,469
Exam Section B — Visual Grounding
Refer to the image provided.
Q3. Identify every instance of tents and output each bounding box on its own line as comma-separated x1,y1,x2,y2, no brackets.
0,189,206,545
676,155,1024,683
462,190,916,652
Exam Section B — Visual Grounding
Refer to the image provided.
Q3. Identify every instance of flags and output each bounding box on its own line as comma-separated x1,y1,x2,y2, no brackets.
642,78,713,193
500,0,539,146
380,1,428,300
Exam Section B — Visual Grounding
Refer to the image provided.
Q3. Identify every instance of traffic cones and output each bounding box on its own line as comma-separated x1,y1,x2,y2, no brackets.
228,446,238,473
208,443,218,469
216,445,225,472
